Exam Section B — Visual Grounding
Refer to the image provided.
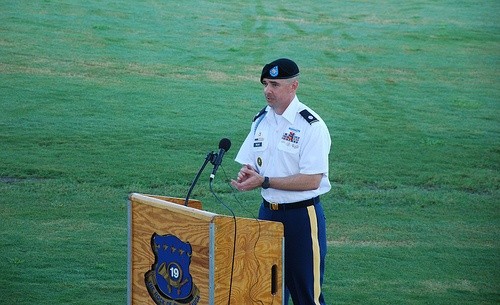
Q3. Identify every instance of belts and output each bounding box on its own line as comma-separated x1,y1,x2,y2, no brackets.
262,195,321,211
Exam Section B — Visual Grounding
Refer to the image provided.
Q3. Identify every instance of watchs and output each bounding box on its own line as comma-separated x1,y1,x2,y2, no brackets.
261,177,270,189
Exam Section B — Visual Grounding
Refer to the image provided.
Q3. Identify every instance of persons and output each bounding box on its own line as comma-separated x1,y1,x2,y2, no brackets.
231,58,332,305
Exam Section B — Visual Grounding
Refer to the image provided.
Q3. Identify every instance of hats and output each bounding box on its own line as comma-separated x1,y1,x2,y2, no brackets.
260,58,300,83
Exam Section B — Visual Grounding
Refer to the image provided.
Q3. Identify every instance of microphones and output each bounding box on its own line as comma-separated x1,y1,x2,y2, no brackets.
210,138,231,180
